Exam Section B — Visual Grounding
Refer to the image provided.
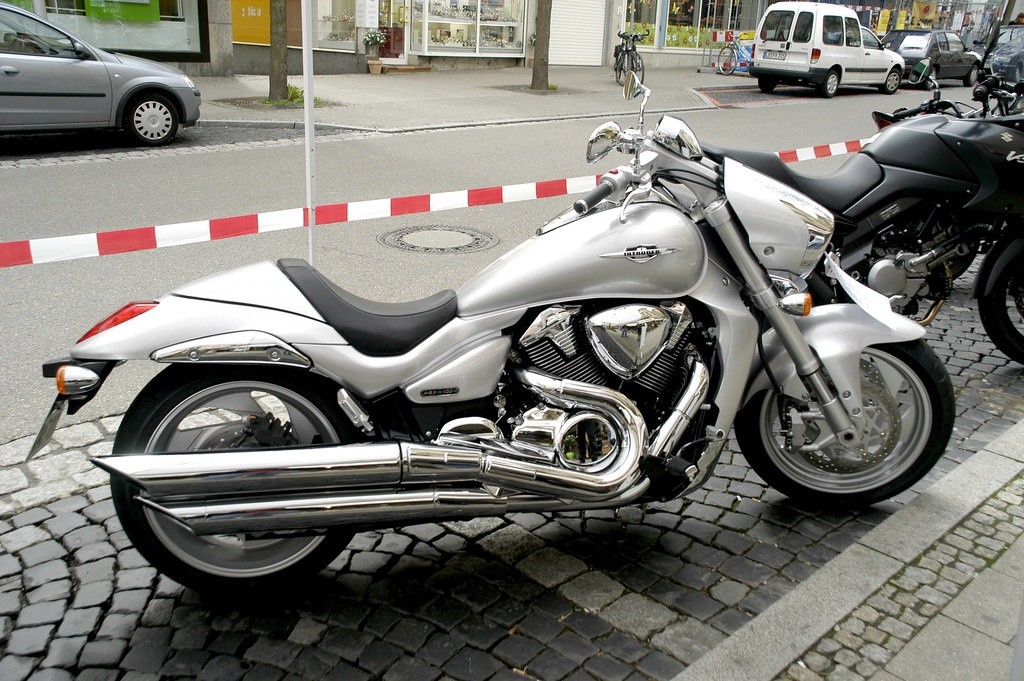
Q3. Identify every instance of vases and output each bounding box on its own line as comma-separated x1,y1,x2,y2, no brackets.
366,59,382,76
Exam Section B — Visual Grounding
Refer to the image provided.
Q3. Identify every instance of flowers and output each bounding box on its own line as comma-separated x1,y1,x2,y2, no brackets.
362,29,389,61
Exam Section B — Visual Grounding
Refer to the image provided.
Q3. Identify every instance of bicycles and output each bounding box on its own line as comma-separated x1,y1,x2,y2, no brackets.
718,32,750,76
614,30,650,86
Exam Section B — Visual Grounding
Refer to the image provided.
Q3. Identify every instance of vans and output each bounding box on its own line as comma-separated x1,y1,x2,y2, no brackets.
749,2,905,98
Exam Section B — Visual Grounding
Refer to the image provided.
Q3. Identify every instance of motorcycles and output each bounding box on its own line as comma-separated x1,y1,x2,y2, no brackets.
699,74,1024,365
26,72,958,601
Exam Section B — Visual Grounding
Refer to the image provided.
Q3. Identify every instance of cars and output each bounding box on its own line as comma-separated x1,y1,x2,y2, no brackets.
972,26,1024,83
0,4,200,147
880,30,983,89
